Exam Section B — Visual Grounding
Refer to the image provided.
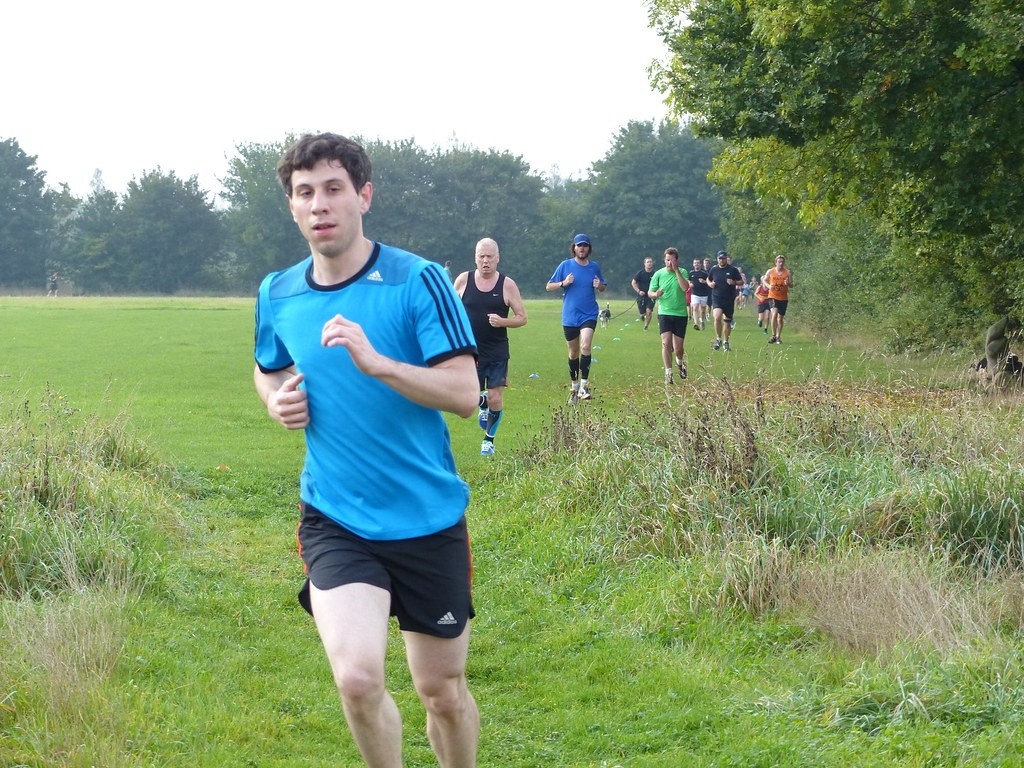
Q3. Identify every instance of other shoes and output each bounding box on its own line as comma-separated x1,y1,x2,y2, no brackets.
680,364,687,379
768,337,781,344
670,374,673,384
764,329,767,334
643,326,648,331
706,314,711,320
731,322,736,329
693,324,700,330
758,321,762,327
723,344,729,349
568,389,579,404
701,321,704,330
713,340,722,349
641,316,645,321
578,382,591,400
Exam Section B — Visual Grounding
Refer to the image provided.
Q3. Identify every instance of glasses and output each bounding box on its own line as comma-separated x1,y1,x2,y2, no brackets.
577,243,588,247
719,258,725,259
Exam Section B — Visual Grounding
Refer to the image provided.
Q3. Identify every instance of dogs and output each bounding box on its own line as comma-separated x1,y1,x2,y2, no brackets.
599,302,610,330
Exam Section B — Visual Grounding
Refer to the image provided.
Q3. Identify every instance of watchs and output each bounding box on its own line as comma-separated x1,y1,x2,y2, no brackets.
559,281,566,289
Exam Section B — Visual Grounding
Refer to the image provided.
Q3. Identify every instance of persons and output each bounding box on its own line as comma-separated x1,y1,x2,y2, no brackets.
443,260,453,284
546,233,608,408
452,236,528,459
630,246,793,335
762,254,793,345
647,247,691,386
46,272,59,298
250,131,484,768
706,250,741,353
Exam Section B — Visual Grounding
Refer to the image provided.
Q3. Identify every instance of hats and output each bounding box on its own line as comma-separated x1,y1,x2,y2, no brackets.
717,251,727,258
574,234,590,244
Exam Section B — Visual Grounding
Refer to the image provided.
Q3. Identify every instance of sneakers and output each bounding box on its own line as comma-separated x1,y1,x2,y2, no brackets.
481,441,494,455
478,391,489,430
